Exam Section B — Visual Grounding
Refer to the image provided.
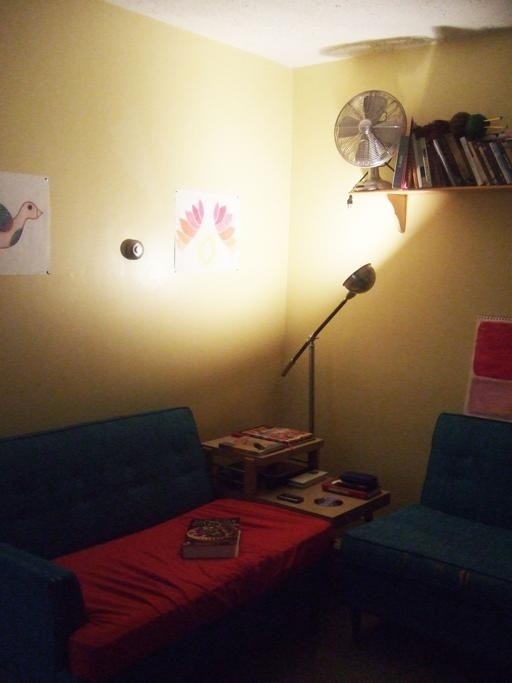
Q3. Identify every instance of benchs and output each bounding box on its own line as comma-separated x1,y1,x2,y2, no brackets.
342,412,511,673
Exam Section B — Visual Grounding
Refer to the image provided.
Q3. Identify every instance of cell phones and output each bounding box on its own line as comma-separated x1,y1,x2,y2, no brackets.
277,493,303,503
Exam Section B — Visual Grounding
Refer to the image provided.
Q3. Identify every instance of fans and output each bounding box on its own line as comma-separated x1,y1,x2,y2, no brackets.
334,90,408,191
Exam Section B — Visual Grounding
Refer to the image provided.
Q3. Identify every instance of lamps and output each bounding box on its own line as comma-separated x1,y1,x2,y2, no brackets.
282,261,377,489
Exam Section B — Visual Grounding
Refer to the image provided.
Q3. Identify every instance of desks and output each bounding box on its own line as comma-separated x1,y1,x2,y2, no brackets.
200,423,390,536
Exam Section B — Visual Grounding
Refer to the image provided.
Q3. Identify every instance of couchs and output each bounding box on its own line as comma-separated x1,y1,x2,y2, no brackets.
0,407,334,683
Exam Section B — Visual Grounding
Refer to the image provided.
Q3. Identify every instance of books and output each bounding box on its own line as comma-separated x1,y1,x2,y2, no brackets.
219,425,329,492
322,470,381,501
392,116,512,190
183,518,239,559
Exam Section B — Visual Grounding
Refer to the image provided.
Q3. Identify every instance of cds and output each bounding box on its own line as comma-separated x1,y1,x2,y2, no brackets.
313,496,344,508
